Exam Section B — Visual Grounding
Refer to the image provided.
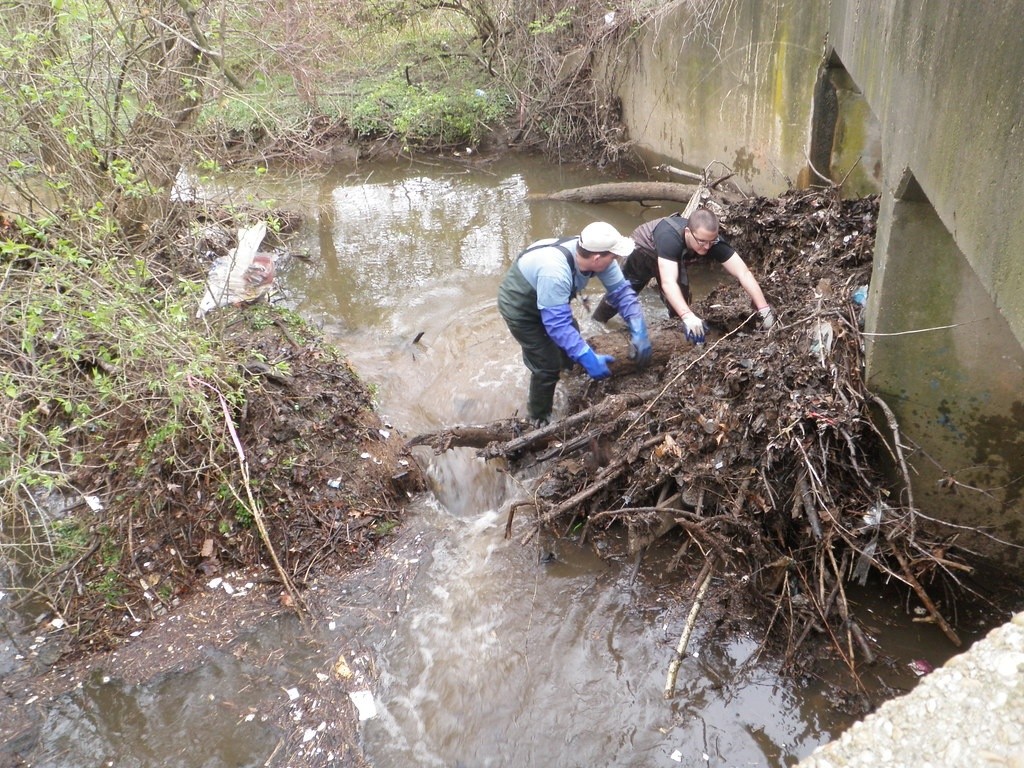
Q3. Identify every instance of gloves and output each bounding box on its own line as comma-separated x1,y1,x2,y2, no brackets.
680,311,711,344
605,281,652,368
759,307,776,331
540,303,616,379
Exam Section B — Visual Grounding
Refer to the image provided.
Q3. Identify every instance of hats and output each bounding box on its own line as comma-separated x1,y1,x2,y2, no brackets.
579,222,635,256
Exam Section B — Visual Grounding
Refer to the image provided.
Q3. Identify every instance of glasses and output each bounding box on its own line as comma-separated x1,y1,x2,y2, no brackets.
690,230,720,244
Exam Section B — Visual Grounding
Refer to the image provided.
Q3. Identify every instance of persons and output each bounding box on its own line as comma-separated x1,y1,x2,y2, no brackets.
592,208,775,341
498,222,653,429
